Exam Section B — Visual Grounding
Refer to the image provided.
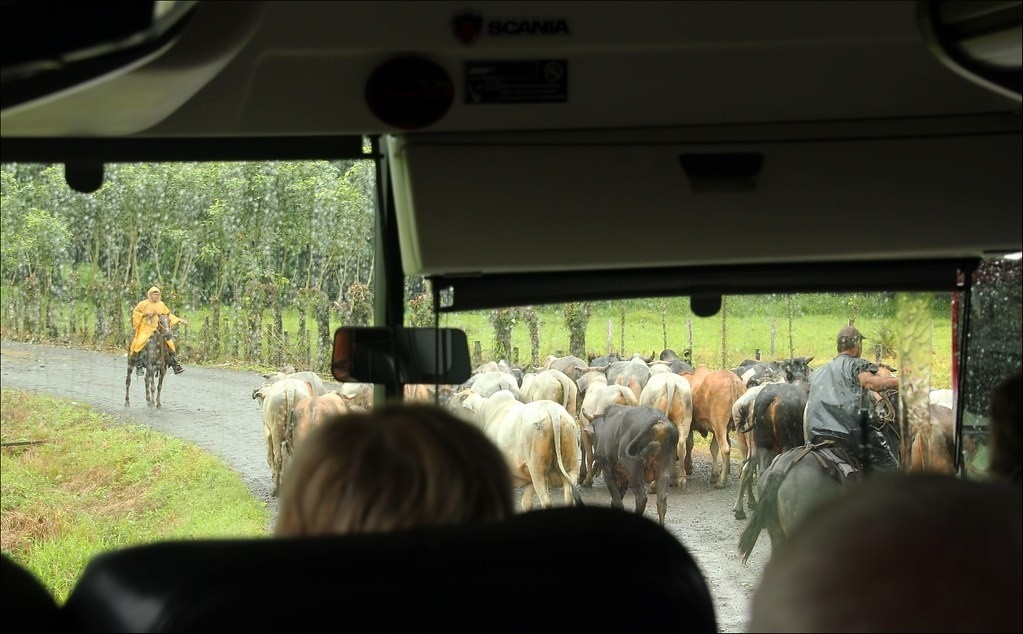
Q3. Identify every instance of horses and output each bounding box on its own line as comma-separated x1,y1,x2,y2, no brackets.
124,311,172,410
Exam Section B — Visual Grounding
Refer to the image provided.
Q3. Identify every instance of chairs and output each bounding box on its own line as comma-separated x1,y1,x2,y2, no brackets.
64,505,720,634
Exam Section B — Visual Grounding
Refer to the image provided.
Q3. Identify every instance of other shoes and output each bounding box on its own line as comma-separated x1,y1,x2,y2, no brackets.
135,366,144,375
175,365,184,373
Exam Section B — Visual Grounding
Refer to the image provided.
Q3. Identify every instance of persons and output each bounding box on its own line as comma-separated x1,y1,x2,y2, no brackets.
749,469,1023,634
129,286,188,374
961,431,990,479
275,402,515,540
803,325,903,478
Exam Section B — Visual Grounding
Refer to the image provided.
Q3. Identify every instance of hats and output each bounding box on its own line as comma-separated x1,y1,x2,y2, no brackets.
837,326,866,342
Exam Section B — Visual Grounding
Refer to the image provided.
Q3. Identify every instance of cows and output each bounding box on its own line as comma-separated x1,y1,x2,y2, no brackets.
680,364,754,488
735,362,910,565
341,382,373,410
616,351,655,389
729,357,815,520
640,361,694,494
901,388,954,476
292,391,358,454
581,403,679,528
262,365,326,395
518,371,578,419
251,378,312,497
660,349,694,373
587,350,651,400
528,354,588,415
575,365,638,487
404,360,584,514
